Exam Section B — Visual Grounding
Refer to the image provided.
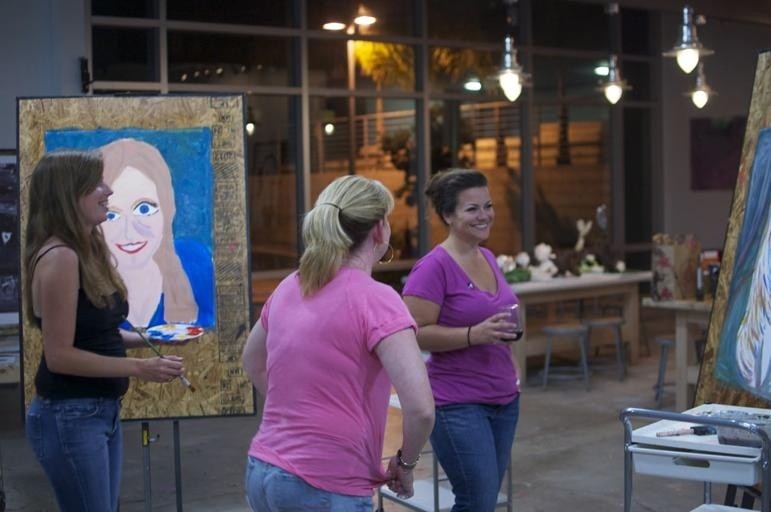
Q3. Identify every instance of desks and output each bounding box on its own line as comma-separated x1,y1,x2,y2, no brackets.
642,294,713,411
508,270,652,385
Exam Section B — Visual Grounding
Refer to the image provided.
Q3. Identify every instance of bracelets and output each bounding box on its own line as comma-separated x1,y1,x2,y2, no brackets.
398,449,420,469
467,325,471,348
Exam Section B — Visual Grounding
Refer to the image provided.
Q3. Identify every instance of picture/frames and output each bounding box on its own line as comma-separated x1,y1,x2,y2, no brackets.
14,93,255,423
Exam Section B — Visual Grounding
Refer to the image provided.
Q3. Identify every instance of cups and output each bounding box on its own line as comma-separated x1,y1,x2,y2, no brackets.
495,302,524,343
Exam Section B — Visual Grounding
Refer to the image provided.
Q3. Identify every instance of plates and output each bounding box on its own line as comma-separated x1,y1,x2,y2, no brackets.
144,322,205,345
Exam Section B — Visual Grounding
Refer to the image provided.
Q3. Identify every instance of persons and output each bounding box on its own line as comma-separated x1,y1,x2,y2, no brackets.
24,151,187,511
94,139,213,330
402,168,521,512
242,173,437,512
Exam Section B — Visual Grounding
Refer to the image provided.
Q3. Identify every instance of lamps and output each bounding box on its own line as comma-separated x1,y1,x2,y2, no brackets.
464,0,717,109
320,1,377,31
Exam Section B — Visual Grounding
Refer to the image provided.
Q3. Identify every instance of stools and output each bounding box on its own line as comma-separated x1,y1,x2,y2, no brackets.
657,333,703,407
541,324,590,391
583,317,628,382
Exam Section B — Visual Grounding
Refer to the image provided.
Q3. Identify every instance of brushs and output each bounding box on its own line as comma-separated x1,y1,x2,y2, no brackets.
656,426,717,437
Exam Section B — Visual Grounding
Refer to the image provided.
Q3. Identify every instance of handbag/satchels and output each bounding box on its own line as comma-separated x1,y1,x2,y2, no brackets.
650,236,700,303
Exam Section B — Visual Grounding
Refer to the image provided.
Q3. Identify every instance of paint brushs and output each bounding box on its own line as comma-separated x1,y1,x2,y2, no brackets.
122,317,196,393
